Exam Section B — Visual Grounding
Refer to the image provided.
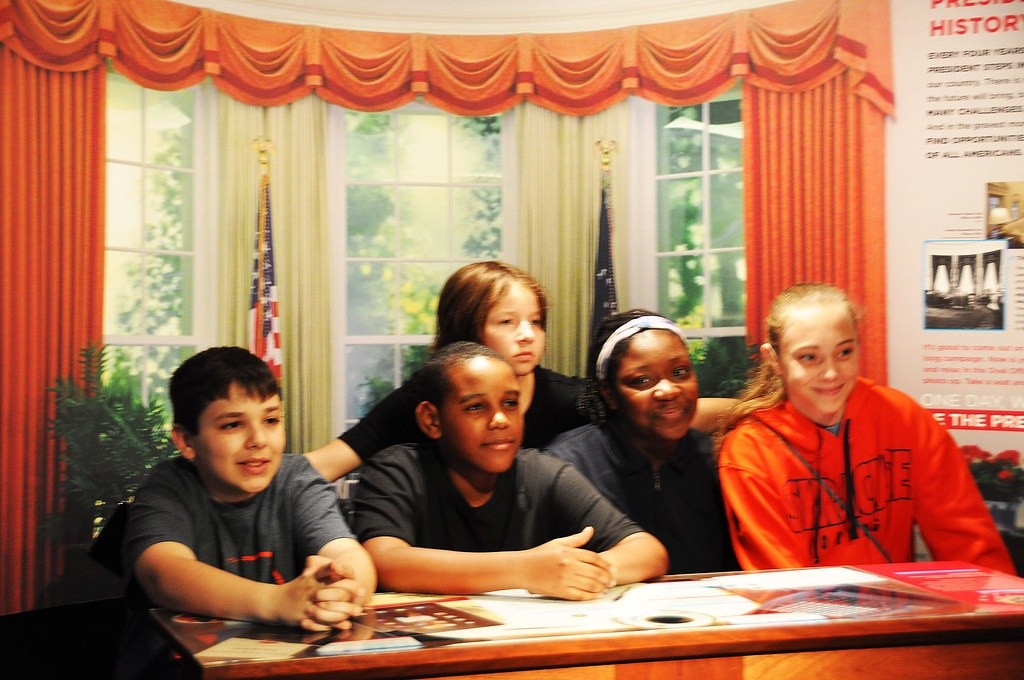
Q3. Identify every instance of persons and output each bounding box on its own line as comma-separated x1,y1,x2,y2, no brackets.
719,280,1018,576
302,261,744,485
349,340,669,602
120,347,377,632
540,311,744,575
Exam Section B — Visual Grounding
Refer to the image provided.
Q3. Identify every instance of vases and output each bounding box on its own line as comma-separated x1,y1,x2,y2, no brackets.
985,501,1018,538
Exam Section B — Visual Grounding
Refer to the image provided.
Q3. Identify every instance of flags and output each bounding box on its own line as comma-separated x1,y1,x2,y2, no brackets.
591,182,619,341
247,177,282,390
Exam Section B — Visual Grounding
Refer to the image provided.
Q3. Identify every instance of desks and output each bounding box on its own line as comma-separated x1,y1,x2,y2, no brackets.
146,558,1024,679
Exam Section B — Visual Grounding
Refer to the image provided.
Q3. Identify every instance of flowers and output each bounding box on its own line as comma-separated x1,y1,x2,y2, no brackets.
960,442,1024,500
